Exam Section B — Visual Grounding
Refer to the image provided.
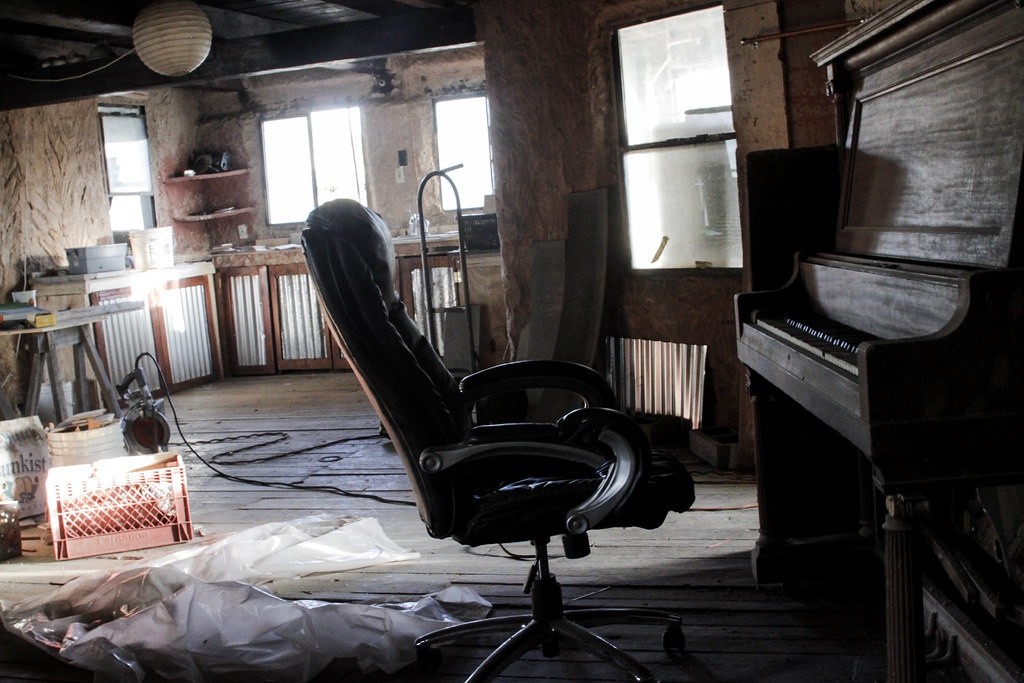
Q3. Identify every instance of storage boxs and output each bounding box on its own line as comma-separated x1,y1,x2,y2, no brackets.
455,214,501,251
65,244,128,273
113,226,173,268
46,451,193,562
0,500,21,562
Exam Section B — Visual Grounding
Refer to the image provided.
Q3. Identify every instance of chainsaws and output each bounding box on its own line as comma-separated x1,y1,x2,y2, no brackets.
114,366,171,452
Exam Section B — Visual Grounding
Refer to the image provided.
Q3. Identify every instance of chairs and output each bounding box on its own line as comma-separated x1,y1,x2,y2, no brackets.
299,199,697,683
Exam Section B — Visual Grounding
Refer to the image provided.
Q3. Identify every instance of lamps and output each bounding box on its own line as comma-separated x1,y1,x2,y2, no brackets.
131,1,211,77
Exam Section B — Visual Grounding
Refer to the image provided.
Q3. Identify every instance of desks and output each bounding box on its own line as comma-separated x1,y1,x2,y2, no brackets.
0,314,122,424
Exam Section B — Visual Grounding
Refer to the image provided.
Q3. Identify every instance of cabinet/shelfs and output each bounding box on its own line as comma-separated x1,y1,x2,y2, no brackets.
33,238,462,400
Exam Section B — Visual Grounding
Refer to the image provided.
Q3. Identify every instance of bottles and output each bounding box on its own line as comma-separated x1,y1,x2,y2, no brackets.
125,239,134,270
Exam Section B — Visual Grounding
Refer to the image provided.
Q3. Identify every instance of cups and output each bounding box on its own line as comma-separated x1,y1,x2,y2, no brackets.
11,289,37,308
409,217,414,236
414,214,419,236
424,220,429,232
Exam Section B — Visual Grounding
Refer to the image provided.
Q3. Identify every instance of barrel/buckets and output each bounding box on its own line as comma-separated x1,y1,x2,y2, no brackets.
46,417,126,466
128,225,175,268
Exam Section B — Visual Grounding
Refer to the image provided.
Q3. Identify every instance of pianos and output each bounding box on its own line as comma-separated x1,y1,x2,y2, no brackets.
731,0,1024,683
